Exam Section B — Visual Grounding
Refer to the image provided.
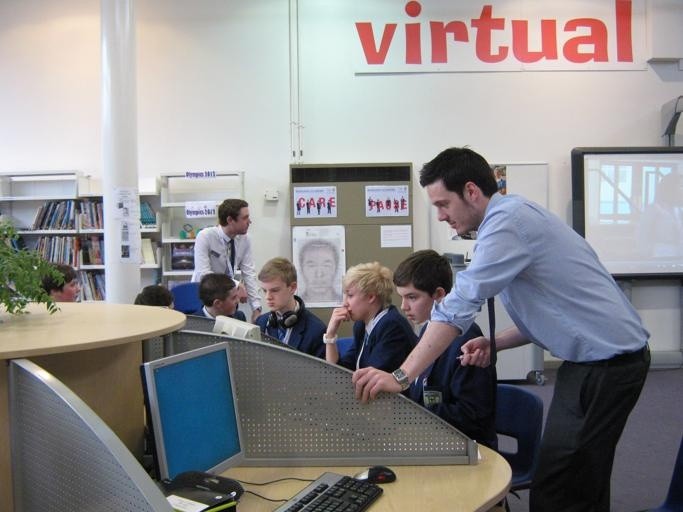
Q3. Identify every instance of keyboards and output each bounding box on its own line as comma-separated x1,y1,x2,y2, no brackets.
272,472,383,511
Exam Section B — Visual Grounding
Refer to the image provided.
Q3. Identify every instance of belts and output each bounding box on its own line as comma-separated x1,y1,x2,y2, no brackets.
574,342,647,368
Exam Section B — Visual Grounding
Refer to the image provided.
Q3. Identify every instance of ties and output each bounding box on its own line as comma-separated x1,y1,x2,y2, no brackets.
230,239,235,273
488,296,496,368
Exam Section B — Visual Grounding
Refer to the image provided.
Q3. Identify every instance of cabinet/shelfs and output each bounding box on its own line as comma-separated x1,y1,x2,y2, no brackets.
0,170,99,303
78,192,161,305
156,170,245,290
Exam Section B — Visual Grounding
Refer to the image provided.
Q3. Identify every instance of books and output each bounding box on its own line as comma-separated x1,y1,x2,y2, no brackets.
76,271,105,301
152,269,162,285
34,236,104,265
4,235,30,255
29,199,103,229
140,201,156,228
142,237,160,265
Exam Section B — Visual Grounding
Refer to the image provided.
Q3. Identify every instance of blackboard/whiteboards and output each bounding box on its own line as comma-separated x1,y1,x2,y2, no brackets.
428,162,549,380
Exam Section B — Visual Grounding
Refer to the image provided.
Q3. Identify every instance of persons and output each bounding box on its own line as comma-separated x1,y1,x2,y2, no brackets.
254,257,327,359
38,263,81,302
351,145,650,512
637,171,682,261
188,273,246,323
323,261,416,399
190,199,262,323
394,250,498,452
299,239,342,303
493,168,506,195
134,286,174,310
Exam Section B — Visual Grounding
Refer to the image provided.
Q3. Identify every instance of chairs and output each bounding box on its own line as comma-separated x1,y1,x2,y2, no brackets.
492,382,544,510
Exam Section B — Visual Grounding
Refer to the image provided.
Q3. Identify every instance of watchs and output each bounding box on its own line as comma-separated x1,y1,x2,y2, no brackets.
392,368,410,391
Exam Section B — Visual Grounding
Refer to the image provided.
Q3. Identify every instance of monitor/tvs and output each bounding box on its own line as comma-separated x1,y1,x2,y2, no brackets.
211,314,262,341
571,146,683,280
139,341,245,485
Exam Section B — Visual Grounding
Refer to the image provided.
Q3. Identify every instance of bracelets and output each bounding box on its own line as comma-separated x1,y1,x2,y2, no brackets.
322,333,338,344
257,306,263,312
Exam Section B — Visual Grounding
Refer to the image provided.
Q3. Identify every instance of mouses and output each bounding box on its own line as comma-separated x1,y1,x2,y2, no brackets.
354,465,396,483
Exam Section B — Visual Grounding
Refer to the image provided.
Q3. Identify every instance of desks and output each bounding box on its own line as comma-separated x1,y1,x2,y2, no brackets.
0,441,515,511
